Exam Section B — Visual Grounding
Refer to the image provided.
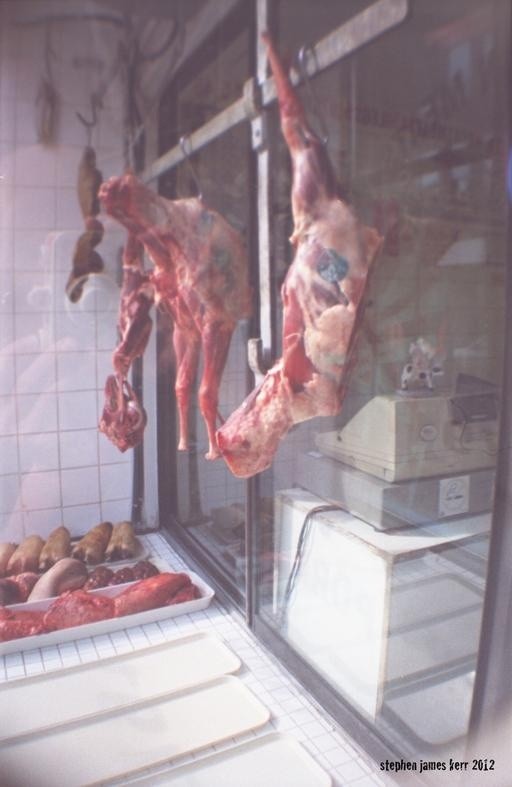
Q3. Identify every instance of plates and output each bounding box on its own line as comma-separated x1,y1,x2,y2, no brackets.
1,539,335,787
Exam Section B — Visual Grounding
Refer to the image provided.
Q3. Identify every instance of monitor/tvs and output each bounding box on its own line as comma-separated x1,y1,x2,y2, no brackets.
450,390,497,423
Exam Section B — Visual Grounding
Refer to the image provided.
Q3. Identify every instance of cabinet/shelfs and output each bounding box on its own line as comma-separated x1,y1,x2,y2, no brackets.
272,488,493,722
